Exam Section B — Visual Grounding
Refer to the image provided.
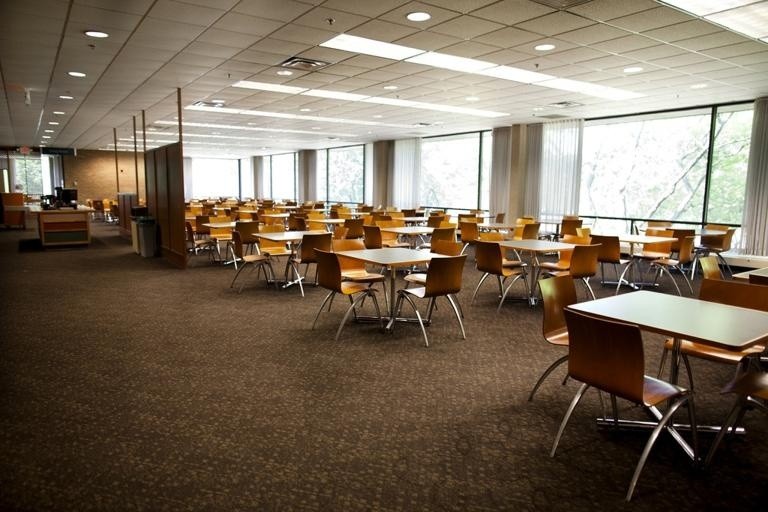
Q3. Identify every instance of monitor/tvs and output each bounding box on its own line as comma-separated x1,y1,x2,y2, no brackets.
59,189,77,207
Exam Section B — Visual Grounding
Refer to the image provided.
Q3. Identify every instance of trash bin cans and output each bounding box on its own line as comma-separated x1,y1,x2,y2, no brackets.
128,216,157,257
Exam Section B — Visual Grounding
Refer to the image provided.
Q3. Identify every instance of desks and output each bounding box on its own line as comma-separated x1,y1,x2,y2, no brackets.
250,229,335,289
487,239,579,312
200,222,264,265
732,265,768,285
333,247,454,333
570,287,768,437
598,233,679,291
27,202,97,247
639,226,727,256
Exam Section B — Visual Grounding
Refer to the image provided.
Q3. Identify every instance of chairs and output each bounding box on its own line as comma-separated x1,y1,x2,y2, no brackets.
230,221,260,257
529,242,603,305
702,370,768,471
634,225,674,273
589,234,639,296
184,221,223,266
290,233,333,287
392,253,467,347
547,304,706,504
699,224,730,245
311,248,385,345
630,228,674,283
527,274,643,422
660,228,698,283
647,220,674,259
533,234,594,303
471,238,532,315
399,239,471,323
361,224,396,278
479,232,532,299
258,225,298,283
656,279,768,429
228,231,278,295
686,229,736,283
86,197,630,237
332,238,392,321
642,235,697,297
